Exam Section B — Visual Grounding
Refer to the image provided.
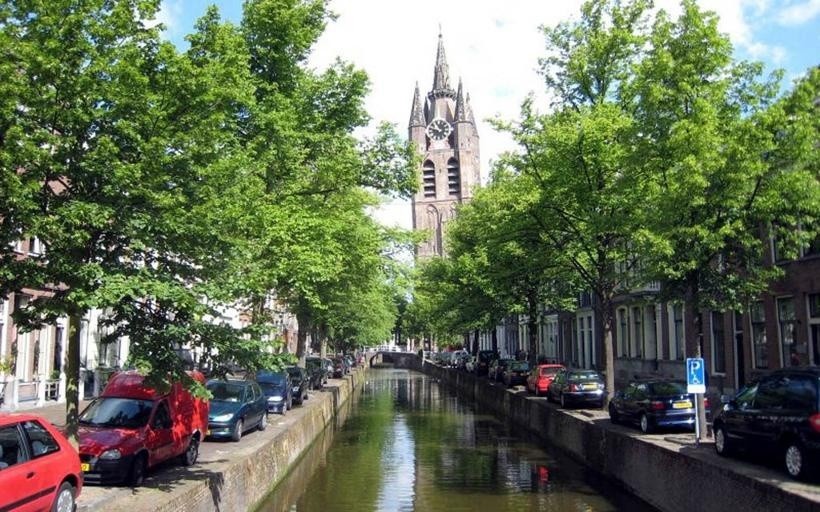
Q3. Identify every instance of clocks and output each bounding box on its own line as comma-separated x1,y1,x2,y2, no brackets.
426,119,451,143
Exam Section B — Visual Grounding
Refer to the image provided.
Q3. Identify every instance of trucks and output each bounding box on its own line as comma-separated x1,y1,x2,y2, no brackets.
76,370,210,485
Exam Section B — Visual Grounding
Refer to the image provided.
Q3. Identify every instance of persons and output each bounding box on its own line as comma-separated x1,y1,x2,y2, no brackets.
515,349,549,365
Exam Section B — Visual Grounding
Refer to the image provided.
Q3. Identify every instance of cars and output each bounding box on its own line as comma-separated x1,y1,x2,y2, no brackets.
287,366,310,406
548,370,606,409
715,368,816,479
437,343,532,387
527,365,562,392
609,377,713,432
255,370,293,414
306,353,365,390
204,377,268,441
1,413,83,512
